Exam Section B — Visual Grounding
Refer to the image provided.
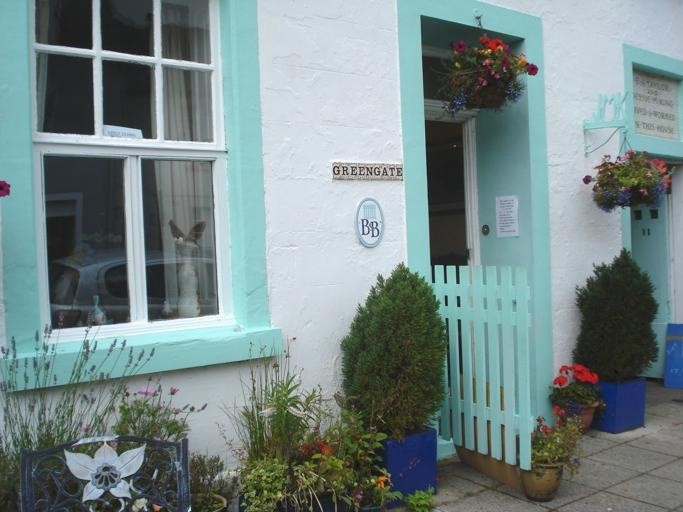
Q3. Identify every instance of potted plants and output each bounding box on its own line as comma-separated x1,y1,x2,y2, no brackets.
567,248,659,435
336,263,450,510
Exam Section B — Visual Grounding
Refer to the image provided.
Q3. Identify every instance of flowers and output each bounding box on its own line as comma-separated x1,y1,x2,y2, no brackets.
582,147,676,211
518,406,584,480
430,31,539,120
548,364,607,419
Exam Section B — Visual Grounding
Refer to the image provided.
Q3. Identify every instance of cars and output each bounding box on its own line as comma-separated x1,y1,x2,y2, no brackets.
43,245,219,330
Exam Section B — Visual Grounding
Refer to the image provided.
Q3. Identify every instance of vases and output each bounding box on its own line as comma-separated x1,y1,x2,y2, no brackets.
519,461,565,503
629,191,654,206
563,401,601,433
465,83,505,108
